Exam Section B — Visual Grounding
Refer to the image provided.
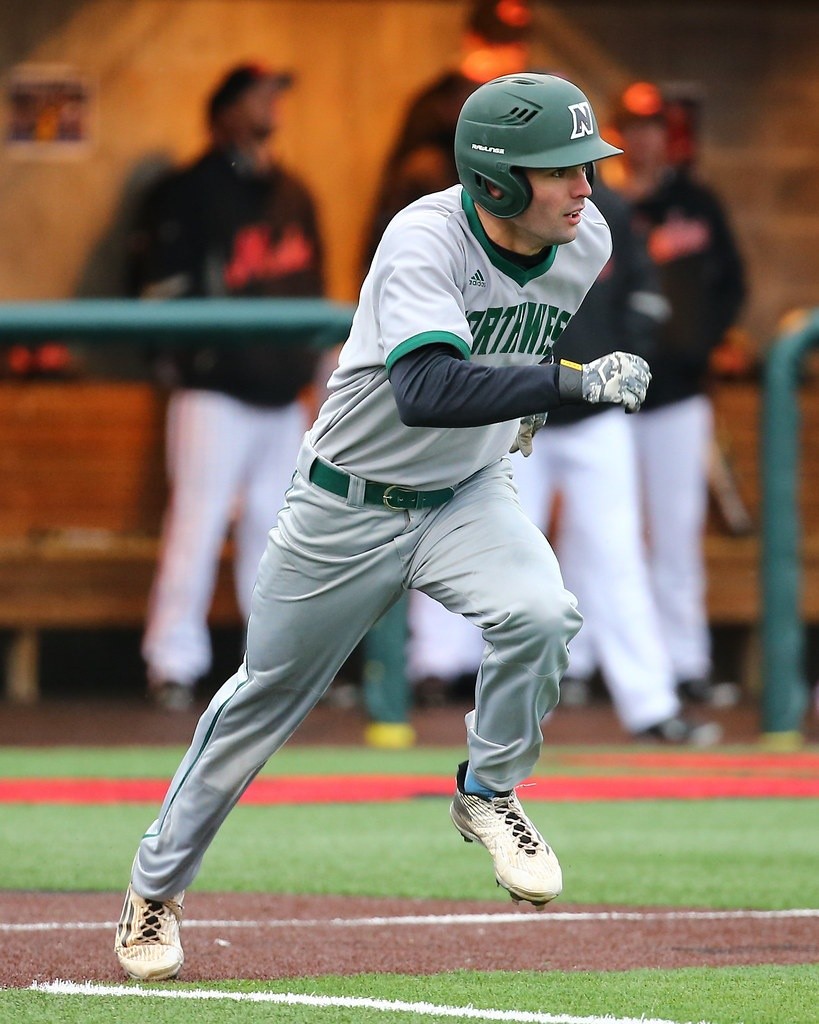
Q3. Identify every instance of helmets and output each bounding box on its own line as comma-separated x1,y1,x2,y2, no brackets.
456,74,625,219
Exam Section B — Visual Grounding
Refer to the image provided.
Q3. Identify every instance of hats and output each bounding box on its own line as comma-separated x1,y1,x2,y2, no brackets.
208,65,294,121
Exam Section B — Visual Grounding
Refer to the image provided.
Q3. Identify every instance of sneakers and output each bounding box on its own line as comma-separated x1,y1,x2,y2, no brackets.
114,881,185,982
450,760,563,907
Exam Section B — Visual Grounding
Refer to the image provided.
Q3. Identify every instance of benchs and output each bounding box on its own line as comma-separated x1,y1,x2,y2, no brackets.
0,374,819,635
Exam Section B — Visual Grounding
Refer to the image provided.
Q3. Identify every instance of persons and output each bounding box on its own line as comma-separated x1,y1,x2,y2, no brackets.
129,59,327,712
352,1,739,747
112,73,650,981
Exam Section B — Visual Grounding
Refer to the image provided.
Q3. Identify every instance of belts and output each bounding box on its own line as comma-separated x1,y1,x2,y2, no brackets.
309,457,458,511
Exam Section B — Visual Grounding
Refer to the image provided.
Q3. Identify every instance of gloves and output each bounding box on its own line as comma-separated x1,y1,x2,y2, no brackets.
509,411,549,457
557,351,653,415
